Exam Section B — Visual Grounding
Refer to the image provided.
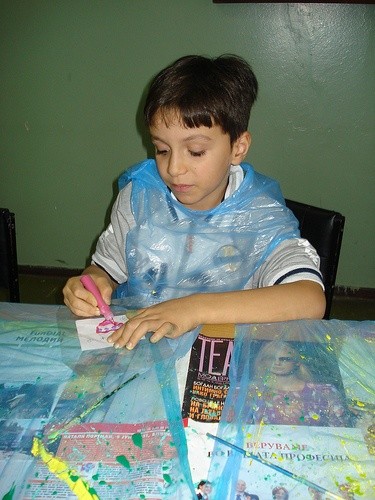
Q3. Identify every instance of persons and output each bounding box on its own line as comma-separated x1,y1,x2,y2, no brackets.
62,53,326,350
228,340,340,425
194,478,291,500
61,353,110,402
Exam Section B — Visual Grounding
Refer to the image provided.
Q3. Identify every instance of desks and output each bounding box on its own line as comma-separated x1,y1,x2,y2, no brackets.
0,302,375,500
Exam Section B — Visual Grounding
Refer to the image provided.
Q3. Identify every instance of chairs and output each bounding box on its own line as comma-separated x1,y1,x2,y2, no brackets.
284,199,347,320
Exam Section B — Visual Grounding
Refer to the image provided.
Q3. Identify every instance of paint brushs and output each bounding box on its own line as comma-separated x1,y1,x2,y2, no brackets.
47,373,140,440
206,432,347,499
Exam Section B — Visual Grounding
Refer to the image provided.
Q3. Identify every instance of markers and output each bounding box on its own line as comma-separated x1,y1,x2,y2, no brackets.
80,274,117,326
